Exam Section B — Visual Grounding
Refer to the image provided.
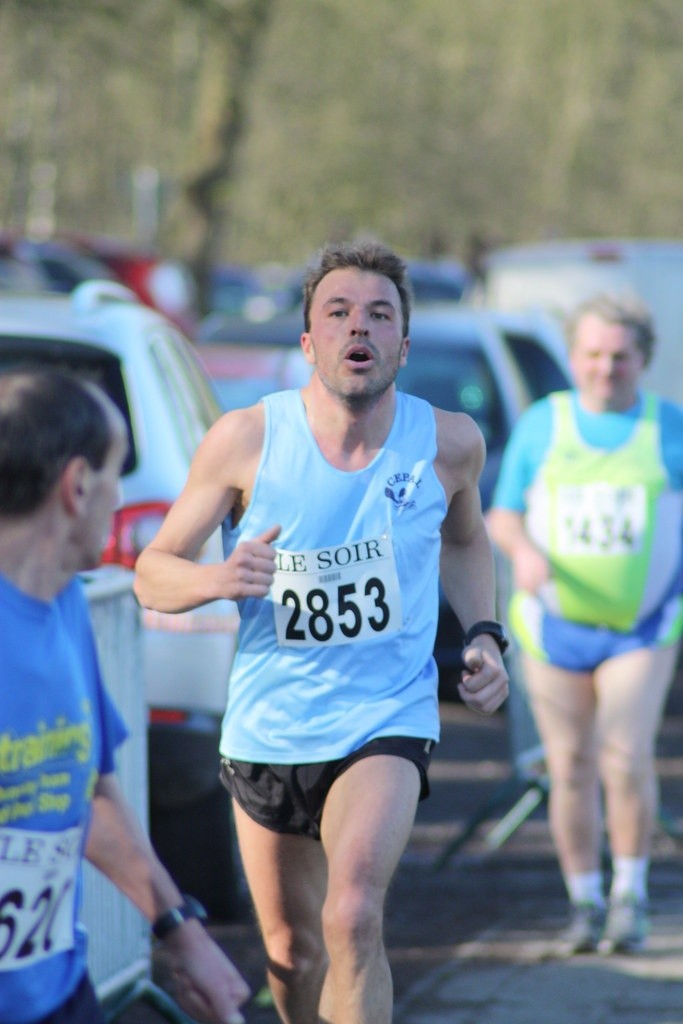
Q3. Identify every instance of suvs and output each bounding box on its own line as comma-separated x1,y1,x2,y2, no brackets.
1,236,683,927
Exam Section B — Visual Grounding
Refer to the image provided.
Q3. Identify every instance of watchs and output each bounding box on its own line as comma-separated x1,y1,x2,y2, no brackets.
150,895,209,940
463,619,510,654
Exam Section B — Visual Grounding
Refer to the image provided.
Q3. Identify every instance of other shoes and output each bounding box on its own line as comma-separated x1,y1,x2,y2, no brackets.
601,896,647,952
562,905,603,953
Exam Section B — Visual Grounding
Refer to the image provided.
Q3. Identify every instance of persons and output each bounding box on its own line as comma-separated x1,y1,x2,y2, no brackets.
1,369,253,1023
132,240,513,1024
483,286,683,959
